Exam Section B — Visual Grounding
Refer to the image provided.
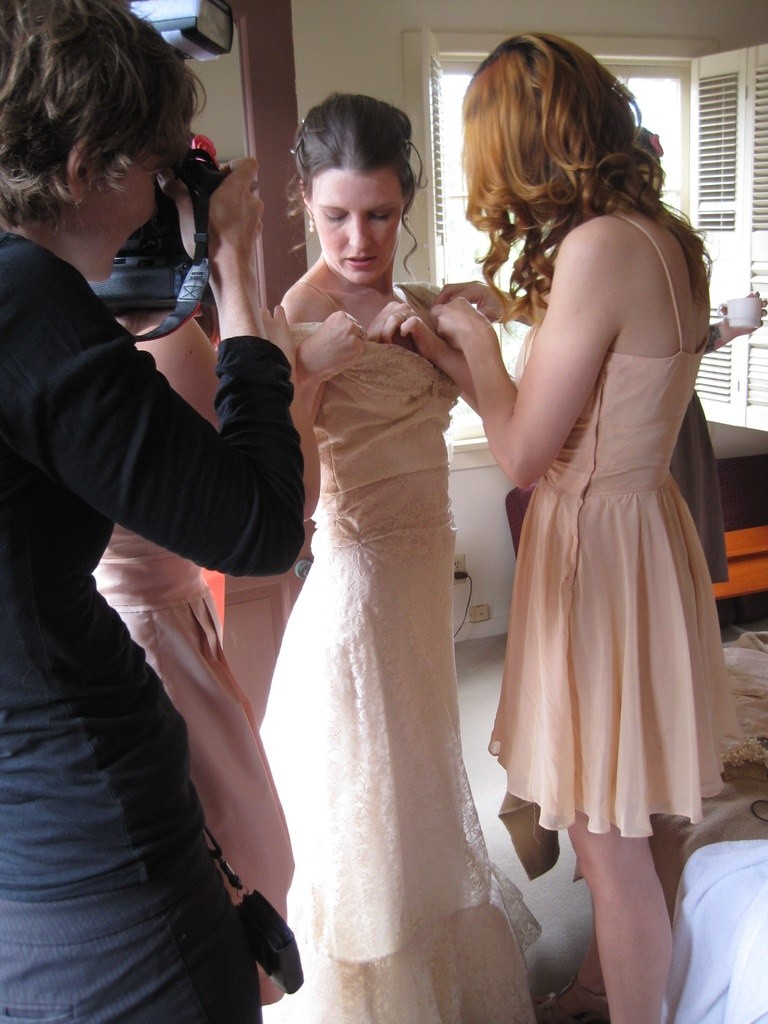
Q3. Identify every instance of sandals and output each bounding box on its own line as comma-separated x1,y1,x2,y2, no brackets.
534,972,610,1024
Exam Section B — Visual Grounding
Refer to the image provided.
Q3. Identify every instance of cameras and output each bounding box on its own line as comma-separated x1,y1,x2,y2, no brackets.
86,149,225,309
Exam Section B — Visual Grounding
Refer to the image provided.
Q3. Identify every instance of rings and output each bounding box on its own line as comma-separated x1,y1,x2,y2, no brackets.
357,325,367,340
399,310,409,321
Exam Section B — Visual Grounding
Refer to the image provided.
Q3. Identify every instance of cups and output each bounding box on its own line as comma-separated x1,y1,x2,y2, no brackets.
717,297,762,329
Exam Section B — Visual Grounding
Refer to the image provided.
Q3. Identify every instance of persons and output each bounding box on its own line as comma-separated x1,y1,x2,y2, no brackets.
430,31,768,1024
0,0,322,1024
255,95,548,1024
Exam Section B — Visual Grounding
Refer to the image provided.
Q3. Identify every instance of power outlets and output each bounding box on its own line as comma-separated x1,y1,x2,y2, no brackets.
452,553,466,584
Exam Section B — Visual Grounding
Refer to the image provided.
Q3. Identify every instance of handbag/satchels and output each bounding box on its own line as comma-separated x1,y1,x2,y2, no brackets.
234,889,304,994
